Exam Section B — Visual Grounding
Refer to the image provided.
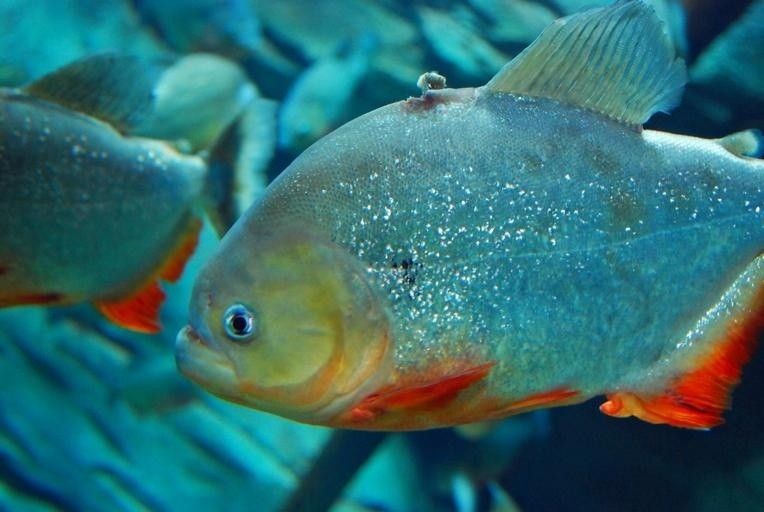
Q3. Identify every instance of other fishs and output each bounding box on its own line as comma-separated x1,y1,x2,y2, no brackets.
0,21,382,339
174,0,763,434
